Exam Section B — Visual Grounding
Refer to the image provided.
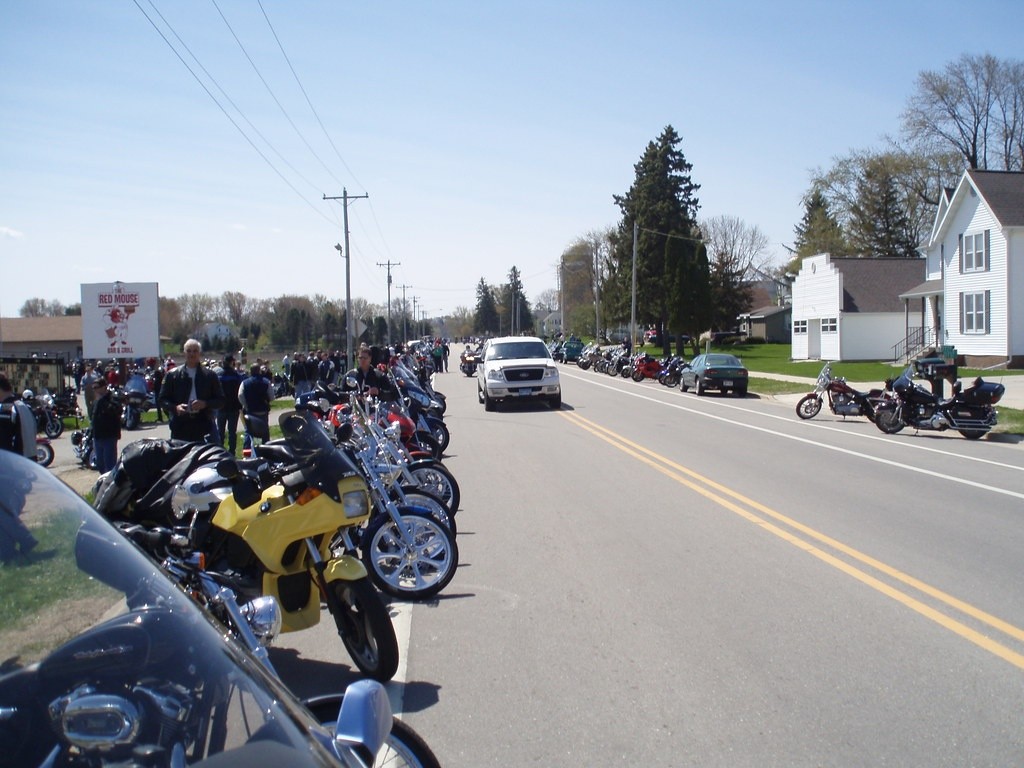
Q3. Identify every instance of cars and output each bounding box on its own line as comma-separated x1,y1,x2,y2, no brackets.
679,353,749,397
644,328,690,345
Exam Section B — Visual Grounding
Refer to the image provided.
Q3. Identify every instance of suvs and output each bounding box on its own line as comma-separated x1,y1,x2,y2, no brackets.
473,335,562,413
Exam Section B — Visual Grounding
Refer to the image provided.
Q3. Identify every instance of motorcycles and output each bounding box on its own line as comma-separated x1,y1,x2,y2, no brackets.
459,352,478,377
873,361,1005,441
0,451,443,768
546,338,688,388
795,359,892,424
0,341,461,683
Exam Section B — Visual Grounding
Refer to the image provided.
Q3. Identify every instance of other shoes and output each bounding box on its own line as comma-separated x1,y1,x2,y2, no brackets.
127,426,133,430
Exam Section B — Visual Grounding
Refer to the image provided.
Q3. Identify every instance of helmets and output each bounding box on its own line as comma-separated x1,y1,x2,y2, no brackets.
22,390,34,400
182,466,235,513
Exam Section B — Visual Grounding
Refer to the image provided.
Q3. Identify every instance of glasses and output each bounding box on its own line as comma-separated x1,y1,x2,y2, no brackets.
359,356,370,361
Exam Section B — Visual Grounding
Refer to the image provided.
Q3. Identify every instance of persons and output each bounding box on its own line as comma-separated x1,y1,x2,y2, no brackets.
559,334,565,344
72,337,407,476
461,345,477,371
0,370,38,562
621,336,631,358
570,333,581,342
421,334,449,373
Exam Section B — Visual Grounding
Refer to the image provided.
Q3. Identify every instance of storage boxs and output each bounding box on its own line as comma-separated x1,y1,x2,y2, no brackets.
973,382,1005,404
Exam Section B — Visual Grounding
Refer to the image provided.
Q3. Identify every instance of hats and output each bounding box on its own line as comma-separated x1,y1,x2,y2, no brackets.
134,369,144,375
85,366,91,371
92,378,106,388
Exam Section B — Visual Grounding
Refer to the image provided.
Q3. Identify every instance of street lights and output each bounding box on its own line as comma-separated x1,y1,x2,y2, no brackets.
334,243,354,371
